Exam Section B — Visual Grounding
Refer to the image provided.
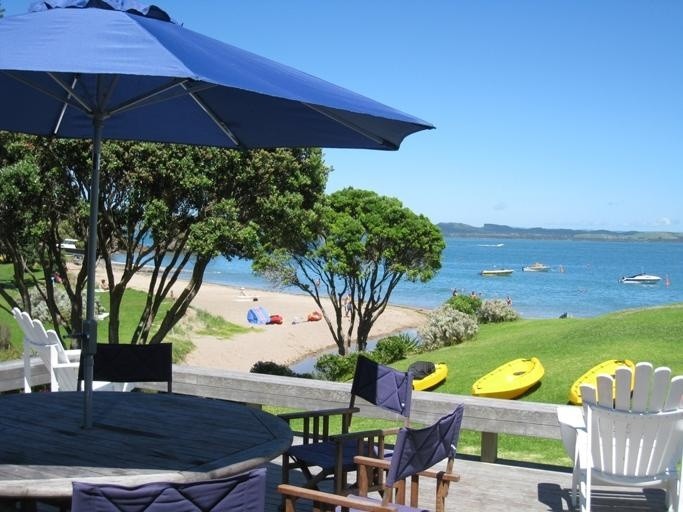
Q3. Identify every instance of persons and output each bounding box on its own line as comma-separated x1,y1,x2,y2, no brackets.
505,295,513,307
343,291,353,317
470,291,477,301
454,289,458,296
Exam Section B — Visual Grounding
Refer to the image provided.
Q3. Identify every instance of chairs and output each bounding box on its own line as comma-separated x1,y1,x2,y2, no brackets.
277,354,415,512
71,468,266,511
278,403,464,512
556,361,683,512
11,306,84,393
76,342,173,394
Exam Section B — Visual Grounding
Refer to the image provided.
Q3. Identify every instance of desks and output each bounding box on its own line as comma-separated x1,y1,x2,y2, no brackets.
0,391,293,512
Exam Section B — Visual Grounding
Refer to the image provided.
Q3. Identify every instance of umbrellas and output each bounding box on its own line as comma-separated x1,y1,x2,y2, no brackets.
0,0,437,427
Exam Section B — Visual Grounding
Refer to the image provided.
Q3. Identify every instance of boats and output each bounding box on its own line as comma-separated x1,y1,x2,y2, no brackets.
520,262,551,272
480,268,515,275
619,272,661,285
566,358,637,407
469,355,546,400
410,361,450,391
56,238,86,254
